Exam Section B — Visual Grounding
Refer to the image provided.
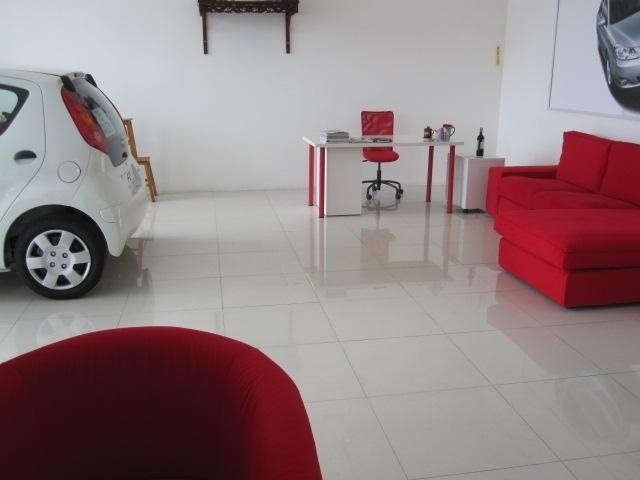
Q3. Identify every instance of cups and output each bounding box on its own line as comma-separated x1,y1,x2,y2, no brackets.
424,126,434,138
443,125,455,139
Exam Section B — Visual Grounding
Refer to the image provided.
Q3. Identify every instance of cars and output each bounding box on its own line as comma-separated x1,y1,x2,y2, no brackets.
596,0,640,108
0,65,152,300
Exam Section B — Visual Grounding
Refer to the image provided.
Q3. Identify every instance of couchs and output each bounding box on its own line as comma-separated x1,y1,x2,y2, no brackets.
485,129,639,310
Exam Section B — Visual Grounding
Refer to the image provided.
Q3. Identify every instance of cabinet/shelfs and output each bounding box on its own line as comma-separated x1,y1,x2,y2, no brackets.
448,154,505,212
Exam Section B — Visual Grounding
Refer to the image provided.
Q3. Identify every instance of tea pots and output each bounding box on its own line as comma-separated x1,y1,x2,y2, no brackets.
435,127,451,142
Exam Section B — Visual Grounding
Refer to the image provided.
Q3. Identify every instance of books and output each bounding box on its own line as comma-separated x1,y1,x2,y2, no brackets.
351,137,372,143
371,137,392,143
320,129,350,144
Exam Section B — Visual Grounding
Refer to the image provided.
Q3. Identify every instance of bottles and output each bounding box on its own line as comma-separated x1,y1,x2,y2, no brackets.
476,127,485,157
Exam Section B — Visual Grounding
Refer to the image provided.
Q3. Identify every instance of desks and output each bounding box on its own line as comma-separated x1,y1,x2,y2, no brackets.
303,136,465,219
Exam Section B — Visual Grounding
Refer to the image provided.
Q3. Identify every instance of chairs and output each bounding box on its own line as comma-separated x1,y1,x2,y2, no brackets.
122,117,159,203
361,110,405,201
0,324,326,479
